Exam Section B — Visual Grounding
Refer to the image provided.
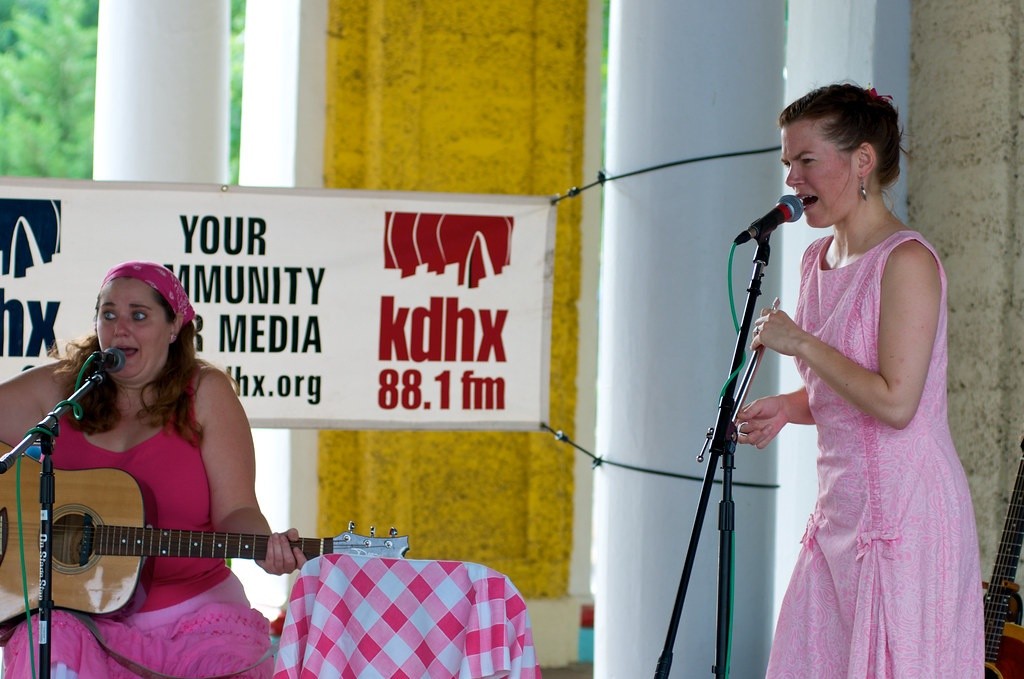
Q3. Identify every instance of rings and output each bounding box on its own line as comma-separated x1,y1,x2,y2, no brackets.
753,327,759,334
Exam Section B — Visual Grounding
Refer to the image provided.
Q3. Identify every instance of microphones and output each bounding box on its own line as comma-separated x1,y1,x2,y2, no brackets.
93,348,126,373
734,195,804,246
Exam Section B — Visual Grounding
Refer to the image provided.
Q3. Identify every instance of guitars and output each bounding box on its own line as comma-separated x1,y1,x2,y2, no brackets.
0,437,412,642
983,435,1024,679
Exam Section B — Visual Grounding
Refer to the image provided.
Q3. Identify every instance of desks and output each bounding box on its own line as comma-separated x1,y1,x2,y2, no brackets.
272,557,546,679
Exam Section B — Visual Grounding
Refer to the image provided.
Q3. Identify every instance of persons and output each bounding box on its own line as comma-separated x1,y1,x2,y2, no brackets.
737,84,985,679
0,262,308,679
